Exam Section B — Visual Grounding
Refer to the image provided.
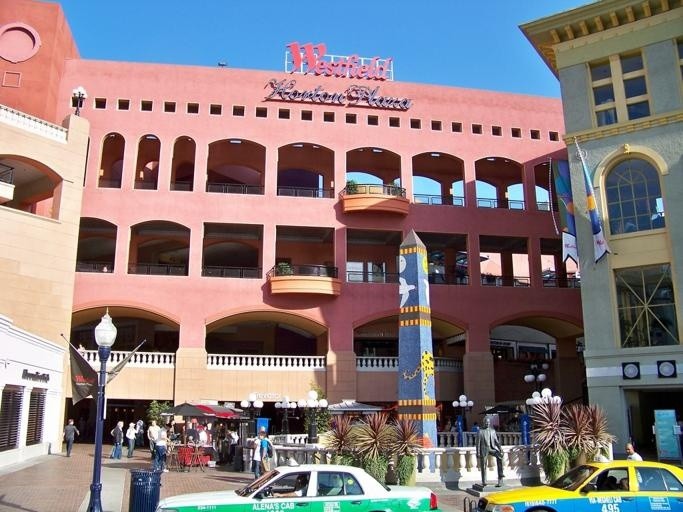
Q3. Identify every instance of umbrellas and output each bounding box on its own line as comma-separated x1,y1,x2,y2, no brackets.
158,401,216,436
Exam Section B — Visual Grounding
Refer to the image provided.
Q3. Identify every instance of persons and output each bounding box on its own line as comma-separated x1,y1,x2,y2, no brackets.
616,478,628,491
61,418,80,458
270,474,307,498
108,417,273,482
474,414,505,485
624,443,644,484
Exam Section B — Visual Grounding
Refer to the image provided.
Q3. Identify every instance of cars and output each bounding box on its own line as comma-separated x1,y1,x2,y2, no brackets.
475,459,683,512
153,457,444,512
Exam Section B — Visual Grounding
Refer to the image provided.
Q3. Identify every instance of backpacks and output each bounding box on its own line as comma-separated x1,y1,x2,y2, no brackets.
261,439,274,458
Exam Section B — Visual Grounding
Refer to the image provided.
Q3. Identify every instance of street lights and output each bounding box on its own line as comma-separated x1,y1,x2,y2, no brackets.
237,389,329,445
522,360,561,406
72,85,87,117
87,304,118,512
449,394,474,433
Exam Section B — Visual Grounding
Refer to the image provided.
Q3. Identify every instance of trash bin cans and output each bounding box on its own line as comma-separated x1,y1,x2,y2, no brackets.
130,469,163,512
179,448,193,473
